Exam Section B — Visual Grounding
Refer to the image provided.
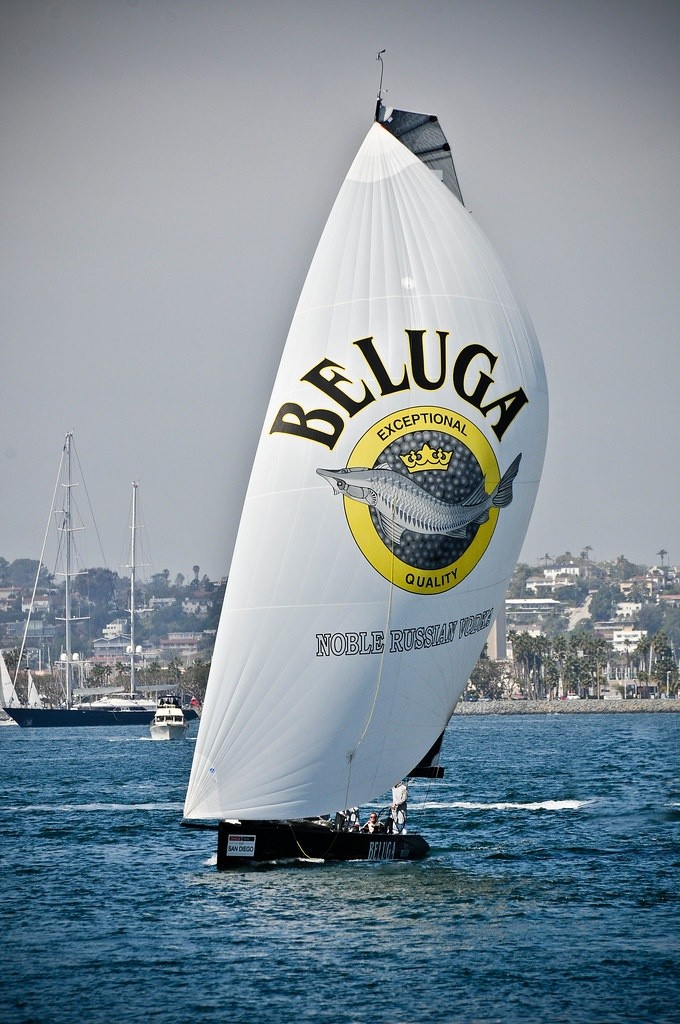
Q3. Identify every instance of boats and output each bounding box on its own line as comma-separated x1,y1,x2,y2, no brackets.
150,691,190,740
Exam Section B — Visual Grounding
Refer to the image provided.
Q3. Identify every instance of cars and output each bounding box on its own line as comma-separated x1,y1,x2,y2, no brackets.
464,695,491,702
566,693,581,700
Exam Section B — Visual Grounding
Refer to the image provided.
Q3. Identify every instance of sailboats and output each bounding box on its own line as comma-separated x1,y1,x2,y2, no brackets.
71,482,157,710
1,431,198,727
177,45,551,876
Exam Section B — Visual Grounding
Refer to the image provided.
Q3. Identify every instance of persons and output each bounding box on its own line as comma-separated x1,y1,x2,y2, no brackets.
160,699,164,705
166,699,171,704
173,699,179,705
392,782,408,835
362,812,384,834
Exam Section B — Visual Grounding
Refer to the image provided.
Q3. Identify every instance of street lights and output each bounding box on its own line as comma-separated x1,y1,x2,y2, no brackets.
667,671,671,697
